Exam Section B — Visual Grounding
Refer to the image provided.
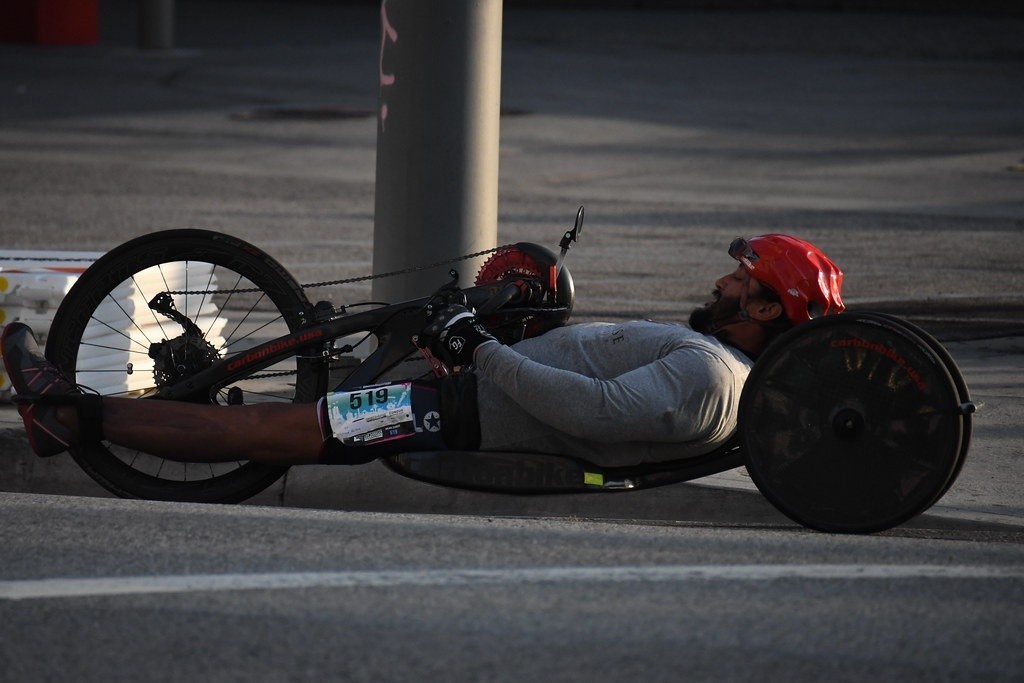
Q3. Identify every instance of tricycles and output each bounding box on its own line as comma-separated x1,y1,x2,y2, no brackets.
7,204,987,535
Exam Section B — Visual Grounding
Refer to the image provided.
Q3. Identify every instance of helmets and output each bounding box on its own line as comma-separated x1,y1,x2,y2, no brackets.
728,234,846,325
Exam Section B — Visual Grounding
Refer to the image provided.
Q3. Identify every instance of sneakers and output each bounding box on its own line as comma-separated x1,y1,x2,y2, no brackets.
0,322,103,457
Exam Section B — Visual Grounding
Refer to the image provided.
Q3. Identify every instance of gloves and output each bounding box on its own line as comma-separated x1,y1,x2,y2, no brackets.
416,303,498,363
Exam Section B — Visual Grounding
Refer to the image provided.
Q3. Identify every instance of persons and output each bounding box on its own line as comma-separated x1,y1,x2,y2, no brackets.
0,235,846,469
